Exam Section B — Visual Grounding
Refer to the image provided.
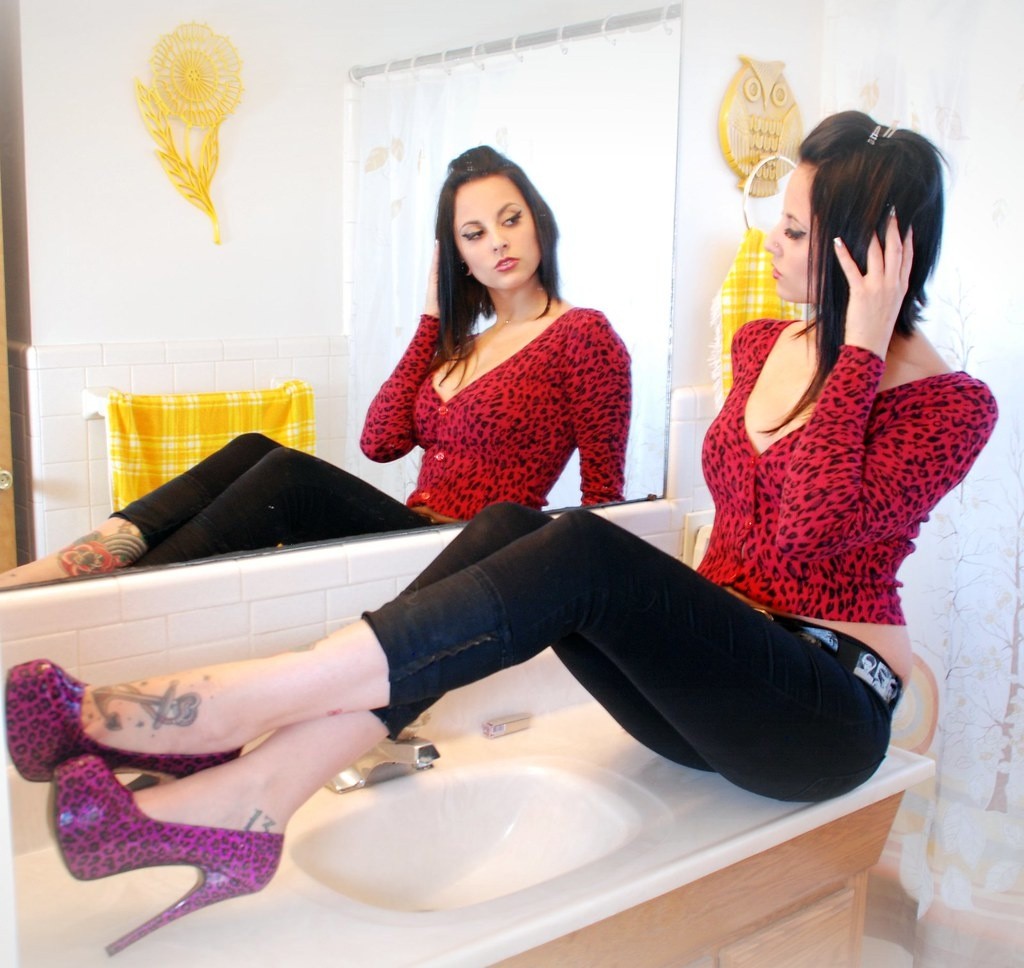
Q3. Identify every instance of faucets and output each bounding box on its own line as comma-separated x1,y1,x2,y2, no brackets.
373,736,439,766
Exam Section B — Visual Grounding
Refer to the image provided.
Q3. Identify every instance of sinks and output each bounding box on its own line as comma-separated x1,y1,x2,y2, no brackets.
287,755,645,918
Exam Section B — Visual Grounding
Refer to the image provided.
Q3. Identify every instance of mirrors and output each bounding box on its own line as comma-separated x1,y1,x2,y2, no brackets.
0,1,680,591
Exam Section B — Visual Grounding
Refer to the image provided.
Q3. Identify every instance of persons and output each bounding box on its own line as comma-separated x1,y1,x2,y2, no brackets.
0,146,635,593
2,111,1004,960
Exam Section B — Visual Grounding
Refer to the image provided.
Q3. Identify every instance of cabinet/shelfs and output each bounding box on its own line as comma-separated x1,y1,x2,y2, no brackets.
484,788,909,968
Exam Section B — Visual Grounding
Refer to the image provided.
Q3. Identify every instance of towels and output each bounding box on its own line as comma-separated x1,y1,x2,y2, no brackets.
107,380,314,515
710,229,801,410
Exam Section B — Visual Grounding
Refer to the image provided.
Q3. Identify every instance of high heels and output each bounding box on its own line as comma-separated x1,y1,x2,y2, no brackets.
5,659,284,956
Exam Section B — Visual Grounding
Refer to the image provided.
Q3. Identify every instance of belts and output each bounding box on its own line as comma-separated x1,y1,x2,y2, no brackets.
787,625,897,705
